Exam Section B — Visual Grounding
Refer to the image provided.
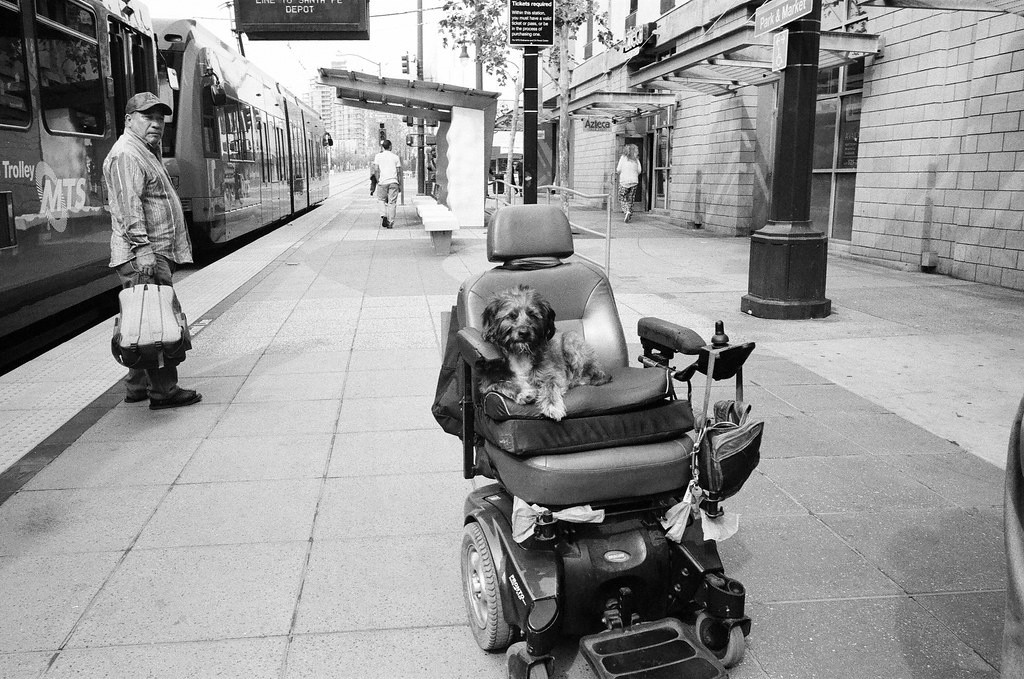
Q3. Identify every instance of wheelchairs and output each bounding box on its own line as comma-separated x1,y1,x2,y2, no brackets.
436,200,758,679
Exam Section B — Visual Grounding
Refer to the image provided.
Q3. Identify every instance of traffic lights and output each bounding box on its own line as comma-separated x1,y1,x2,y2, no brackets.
406,136,413,146
378,128,386,146
402,54,409,75
402,116,413,126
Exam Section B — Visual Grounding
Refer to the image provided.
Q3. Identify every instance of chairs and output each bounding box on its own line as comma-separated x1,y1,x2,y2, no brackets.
456,205,707,506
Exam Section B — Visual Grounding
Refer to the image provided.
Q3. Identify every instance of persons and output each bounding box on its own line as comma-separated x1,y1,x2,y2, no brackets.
616,144,642,222
374,139,402,230
102,92,202,411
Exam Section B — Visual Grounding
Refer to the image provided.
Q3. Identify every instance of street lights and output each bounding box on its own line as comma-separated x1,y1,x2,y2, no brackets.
336,52,382,78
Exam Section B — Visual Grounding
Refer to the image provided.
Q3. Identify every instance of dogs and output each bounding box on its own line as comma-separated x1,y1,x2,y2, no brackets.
476,284,612,422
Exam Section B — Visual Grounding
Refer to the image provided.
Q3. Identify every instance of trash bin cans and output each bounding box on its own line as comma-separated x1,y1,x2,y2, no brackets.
424,179,437,199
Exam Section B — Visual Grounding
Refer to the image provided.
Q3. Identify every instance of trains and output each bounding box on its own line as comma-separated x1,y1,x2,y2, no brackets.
0,0,335,375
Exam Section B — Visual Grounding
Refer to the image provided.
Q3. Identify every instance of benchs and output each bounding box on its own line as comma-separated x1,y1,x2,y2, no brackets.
411,196,460,255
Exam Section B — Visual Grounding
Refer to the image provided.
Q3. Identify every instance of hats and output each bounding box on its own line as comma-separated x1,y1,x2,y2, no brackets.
125,91,173,116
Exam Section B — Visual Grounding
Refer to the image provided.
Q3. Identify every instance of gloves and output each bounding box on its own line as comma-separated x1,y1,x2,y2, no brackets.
135,243,157,274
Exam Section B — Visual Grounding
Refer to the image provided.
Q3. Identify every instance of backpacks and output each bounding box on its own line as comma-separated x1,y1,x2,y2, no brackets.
110,271,192,370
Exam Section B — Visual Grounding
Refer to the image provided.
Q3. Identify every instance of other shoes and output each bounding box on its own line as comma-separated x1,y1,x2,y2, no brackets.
381,215,388,225
624,212,632,223
149,388,202,409
124,396,145,401
389,222,394,229
